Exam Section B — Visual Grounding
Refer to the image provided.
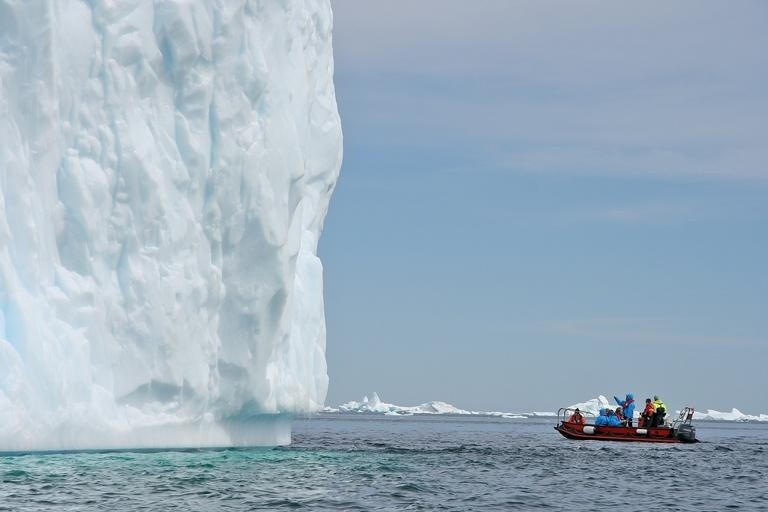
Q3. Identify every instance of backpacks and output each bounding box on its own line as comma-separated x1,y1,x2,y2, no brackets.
657,407,665,418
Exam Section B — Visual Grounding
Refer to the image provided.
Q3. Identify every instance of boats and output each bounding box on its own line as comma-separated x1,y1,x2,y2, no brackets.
554,406,696,443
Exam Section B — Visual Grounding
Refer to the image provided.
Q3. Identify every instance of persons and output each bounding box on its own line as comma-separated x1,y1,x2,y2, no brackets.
613,406,628,427
612,391,635,428
608,406,625,428
651,394,666,428
568,408,585,432
592,407,608,433
638,397,654,428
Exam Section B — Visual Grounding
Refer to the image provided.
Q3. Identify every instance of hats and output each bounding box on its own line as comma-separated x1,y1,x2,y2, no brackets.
646,399,651,403
654,395,659,400
575,408,579,411
